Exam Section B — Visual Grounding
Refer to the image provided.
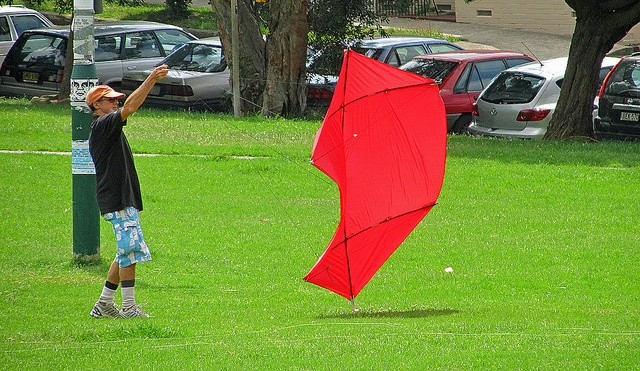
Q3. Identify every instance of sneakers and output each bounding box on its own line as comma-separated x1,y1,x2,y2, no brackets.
90,302,119,318
119,305,149,318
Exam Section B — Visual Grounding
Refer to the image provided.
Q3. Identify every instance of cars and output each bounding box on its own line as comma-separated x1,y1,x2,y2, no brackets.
305,37,465,108
398,49,536,135
121,36,320,111
468,55,622,141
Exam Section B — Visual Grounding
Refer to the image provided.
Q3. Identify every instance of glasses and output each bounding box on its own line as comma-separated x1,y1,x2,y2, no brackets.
96,97,119,103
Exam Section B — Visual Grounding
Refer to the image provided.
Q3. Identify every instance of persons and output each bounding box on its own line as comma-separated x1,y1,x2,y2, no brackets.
85,64,168,318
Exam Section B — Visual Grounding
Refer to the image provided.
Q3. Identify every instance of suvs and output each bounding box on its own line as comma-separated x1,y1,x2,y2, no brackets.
594,51,640,140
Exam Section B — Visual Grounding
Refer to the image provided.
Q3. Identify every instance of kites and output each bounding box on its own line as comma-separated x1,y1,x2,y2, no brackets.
301,47,447,309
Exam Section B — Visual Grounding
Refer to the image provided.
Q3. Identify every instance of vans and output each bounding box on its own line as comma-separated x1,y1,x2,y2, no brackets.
0,4,55,67
0,20,200,98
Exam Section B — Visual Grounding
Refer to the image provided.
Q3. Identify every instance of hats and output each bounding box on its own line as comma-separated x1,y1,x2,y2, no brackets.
86,86,126,104
